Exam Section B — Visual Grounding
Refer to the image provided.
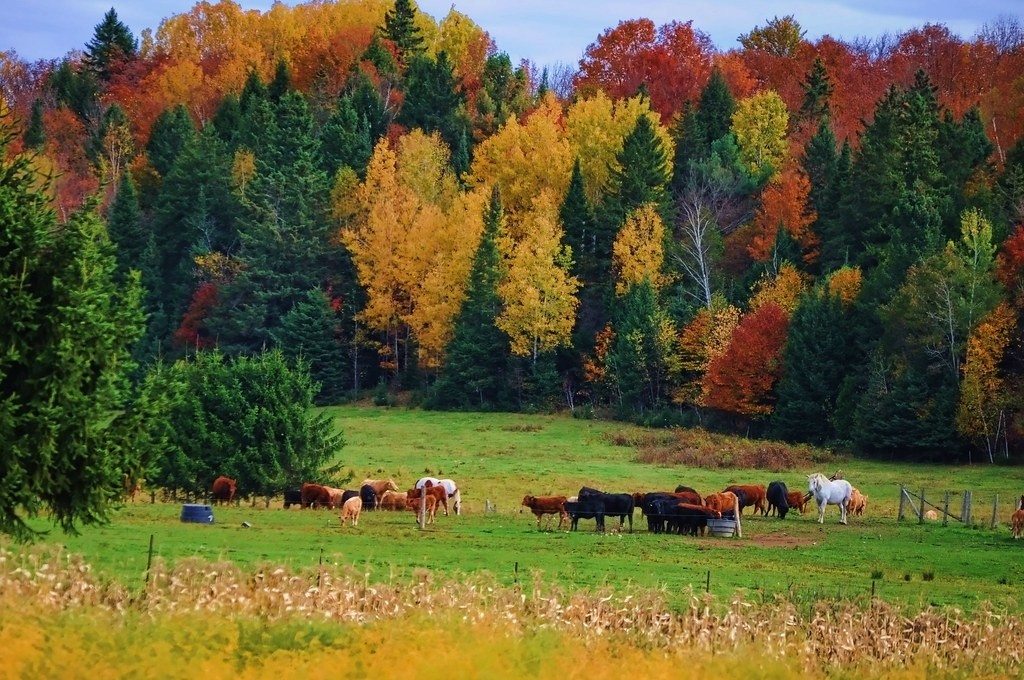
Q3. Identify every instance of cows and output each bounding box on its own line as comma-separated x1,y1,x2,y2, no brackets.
522,481,804,539
210,476,236,507
1009,494,1024,539
283,479,448,528
846,486,868,516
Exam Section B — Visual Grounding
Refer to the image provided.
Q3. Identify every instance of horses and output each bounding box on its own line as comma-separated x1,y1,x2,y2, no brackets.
805,472,852,525
415,477,462,516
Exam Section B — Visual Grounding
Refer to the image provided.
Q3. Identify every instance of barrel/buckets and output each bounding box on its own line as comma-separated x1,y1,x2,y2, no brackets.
180,503,215,525
707,518,736,537
